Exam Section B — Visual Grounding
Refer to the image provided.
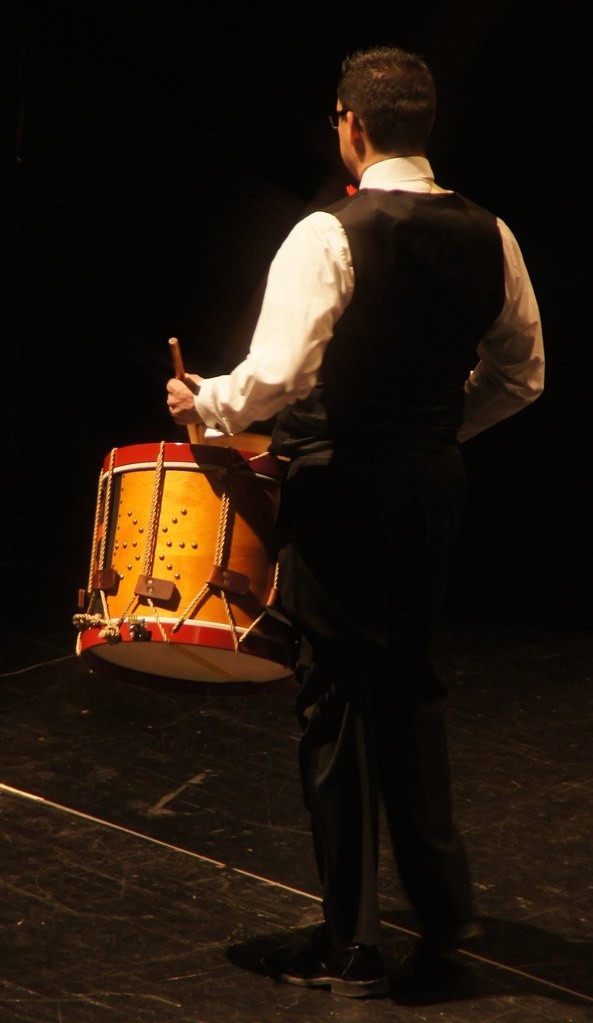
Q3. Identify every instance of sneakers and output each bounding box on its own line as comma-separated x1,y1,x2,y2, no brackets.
388,944,458,990
281,948,377,998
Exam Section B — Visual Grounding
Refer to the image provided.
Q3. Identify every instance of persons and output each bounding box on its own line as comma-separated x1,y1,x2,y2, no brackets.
166,48,545,998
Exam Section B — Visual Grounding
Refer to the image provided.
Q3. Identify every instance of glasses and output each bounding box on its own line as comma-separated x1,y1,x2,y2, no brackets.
326,110,362,130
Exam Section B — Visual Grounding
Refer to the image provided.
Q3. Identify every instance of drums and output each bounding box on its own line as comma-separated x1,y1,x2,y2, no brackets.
75,442,303,697
